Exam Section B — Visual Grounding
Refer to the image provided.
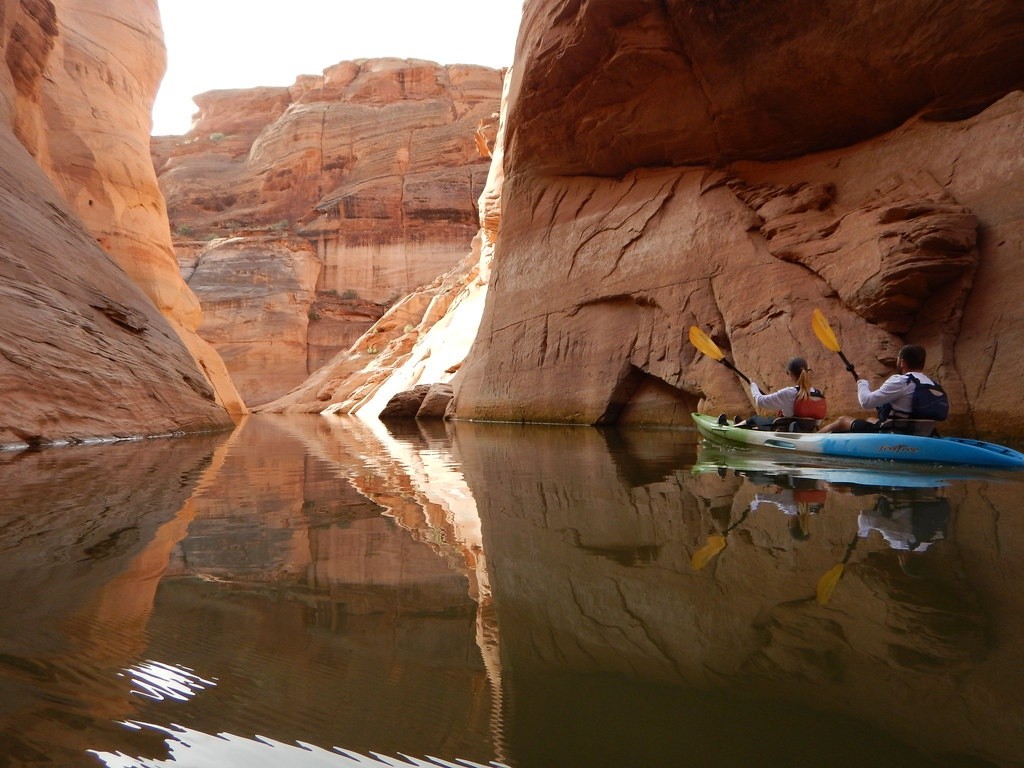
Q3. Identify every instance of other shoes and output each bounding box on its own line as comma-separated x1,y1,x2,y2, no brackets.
718,414,729,426
789,422,802,433
734,415,743,424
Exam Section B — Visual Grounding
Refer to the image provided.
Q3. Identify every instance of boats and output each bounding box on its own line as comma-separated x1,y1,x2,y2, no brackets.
690,411,1024,468
689,443,1023,486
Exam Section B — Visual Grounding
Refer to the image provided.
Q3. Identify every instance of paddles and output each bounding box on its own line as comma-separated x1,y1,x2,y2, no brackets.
692,509,751,569
688,325,767,396
816,500,880,606
810,307,880,411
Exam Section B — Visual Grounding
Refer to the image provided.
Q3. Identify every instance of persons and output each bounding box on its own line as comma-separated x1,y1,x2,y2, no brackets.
716,356,822,433
816,344,935,437
748,487,815,541
853,492,947,580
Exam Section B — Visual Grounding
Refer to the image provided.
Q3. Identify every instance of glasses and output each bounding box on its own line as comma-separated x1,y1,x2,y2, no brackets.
786,370,791,375
